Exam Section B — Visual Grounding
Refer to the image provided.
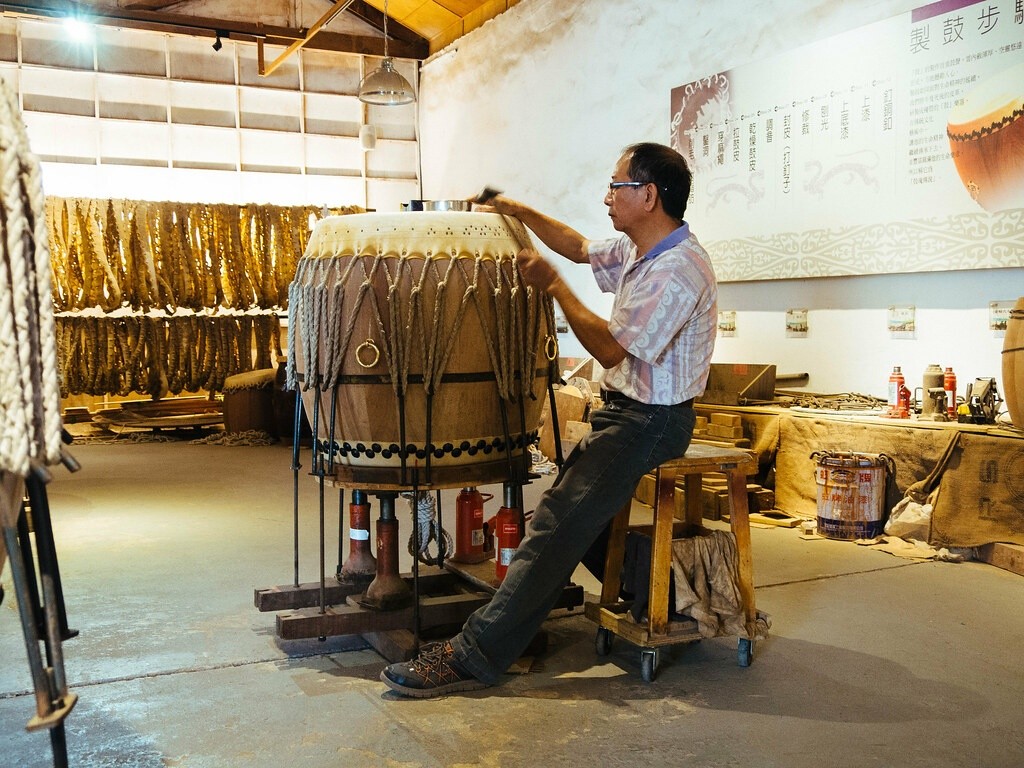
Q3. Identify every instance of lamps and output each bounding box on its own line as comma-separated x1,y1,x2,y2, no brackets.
359,0,416,107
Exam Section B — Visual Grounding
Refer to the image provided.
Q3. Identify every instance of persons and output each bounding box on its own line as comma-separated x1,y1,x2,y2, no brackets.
380,143,718,699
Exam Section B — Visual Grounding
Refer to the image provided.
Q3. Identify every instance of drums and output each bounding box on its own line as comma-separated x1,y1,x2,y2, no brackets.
289,210,557,482
1001,296,1024,430
945,63,1024,212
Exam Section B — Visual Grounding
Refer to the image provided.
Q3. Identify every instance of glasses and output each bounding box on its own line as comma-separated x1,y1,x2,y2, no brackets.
607,181,667,198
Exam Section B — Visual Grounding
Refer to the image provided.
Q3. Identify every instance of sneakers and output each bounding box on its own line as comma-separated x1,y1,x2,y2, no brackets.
619,530,651,602
379,641,492,698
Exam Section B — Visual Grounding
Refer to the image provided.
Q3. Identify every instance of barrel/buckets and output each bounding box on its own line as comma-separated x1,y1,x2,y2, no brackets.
814,450,888,540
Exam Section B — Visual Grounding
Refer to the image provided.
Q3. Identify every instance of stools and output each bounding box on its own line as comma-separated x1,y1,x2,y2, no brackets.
584,442,768,684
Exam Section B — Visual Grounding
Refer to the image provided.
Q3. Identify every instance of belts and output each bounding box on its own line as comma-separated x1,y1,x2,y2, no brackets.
598,387,626,402
